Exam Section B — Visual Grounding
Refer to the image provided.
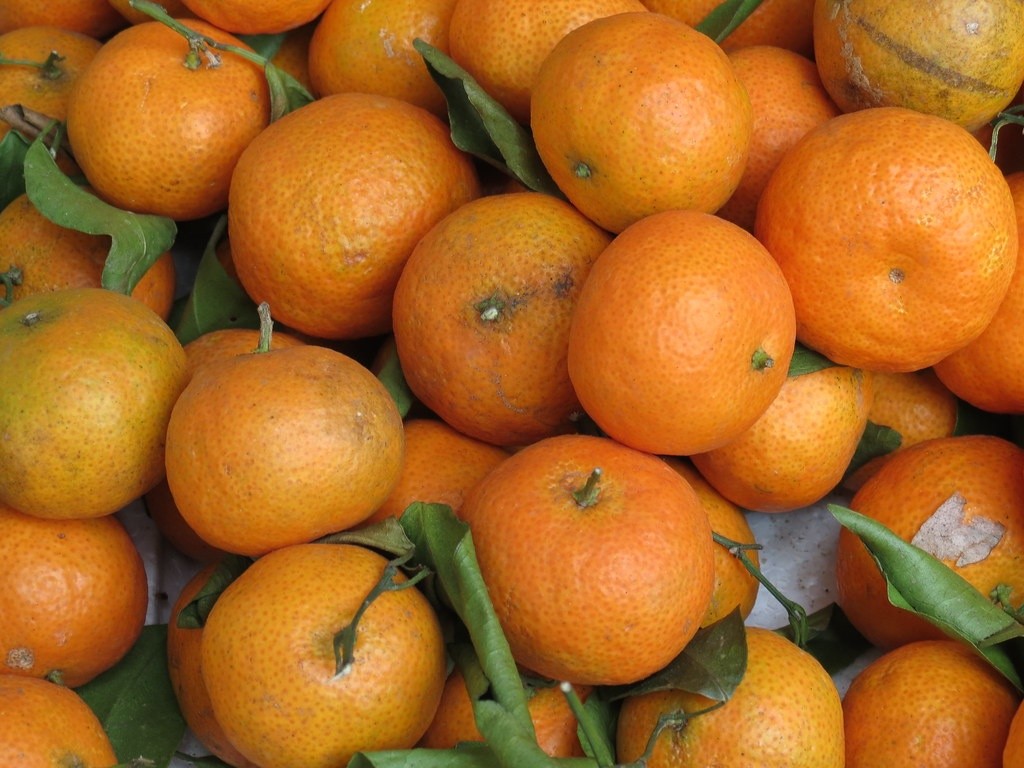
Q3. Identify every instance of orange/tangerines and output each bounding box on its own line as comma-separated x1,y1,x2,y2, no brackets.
1,1,1024,768
